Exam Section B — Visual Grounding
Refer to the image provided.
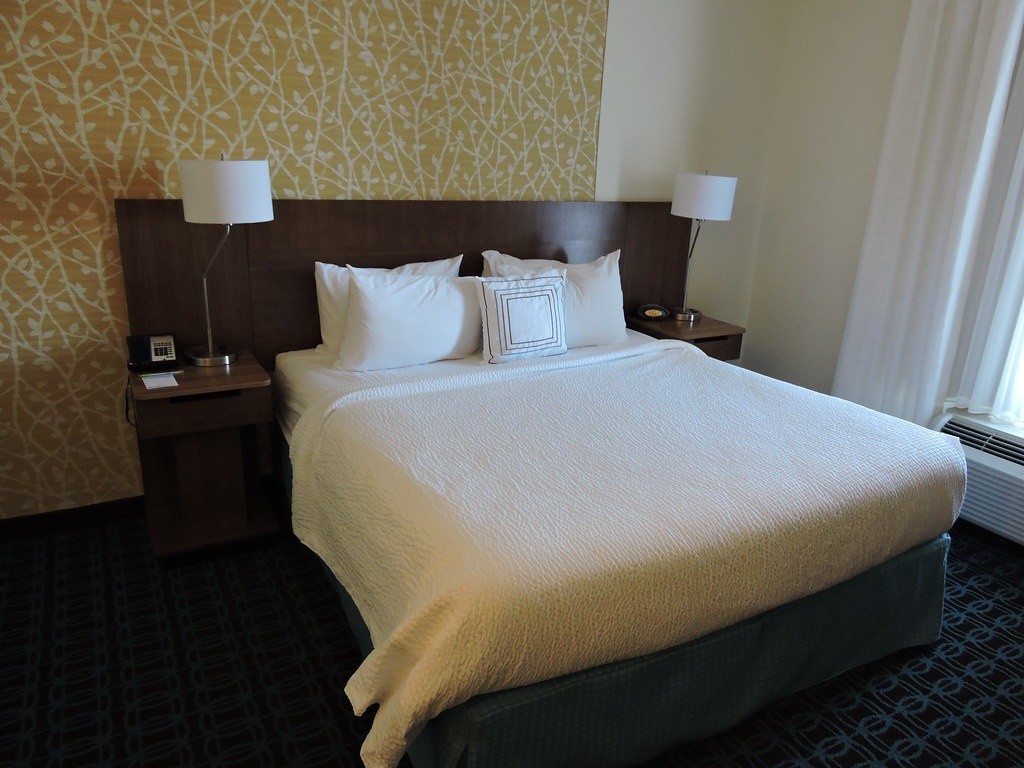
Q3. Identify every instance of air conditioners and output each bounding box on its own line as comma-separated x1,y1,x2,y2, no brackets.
927,411,1024,549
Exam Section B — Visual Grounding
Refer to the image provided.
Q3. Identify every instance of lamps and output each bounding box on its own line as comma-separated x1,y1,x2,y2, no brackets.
668,177,736,325
181,157,274,365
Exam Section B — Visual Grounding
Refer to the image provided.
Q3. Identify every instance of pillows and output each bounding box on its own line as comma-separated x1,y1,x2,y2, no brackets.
472,270,573,362
314,255,462,351
334,265,485,373
486,249,625,350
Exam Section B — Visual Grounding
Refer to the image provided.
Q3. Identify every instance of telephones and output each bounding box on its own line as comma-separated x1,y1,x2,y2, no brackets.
127,333,182,373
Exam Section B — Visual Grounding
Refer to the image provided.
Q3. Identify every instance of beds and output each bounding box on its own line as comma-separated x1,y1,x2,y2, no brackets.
116,200,966,768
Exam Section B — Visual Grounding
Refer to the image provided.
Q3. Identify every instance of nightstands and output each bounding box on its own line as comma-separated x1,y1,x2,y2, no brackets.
640,312,748,360
130,355,279,550
